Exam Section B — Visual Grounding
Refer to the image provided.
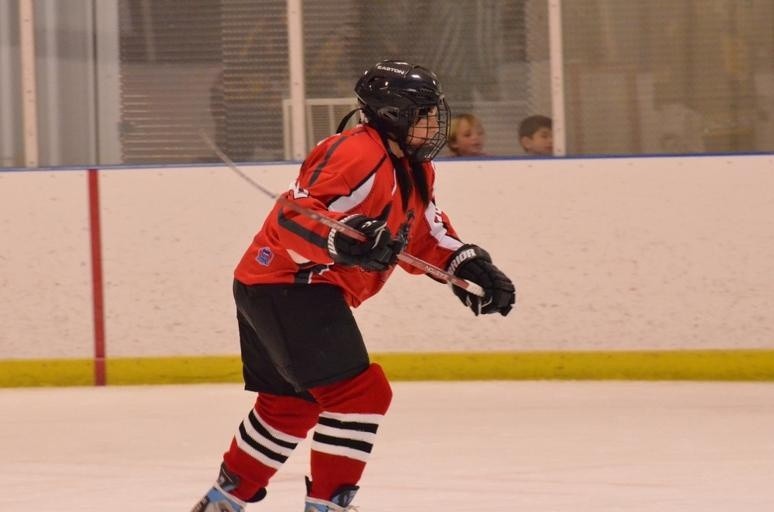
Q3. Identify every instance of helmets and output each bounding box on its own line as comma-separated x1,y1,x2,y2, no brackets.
354,58,445,127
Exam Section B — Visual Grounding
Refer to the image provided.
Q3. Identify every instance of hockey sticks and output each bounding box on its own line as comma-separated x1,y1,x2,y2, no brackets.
200,130,486,299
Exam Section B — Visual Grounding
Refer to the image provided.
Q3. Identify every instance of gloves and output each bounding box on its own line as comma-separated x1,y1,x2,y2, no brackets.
445,243,516,317
325,212,402,273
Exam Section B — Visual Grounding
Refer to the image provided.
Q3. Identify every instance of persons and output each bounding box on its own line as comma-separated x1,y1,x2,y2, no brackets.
519,114,553,155
446,110,491,156
190,58,517,512
